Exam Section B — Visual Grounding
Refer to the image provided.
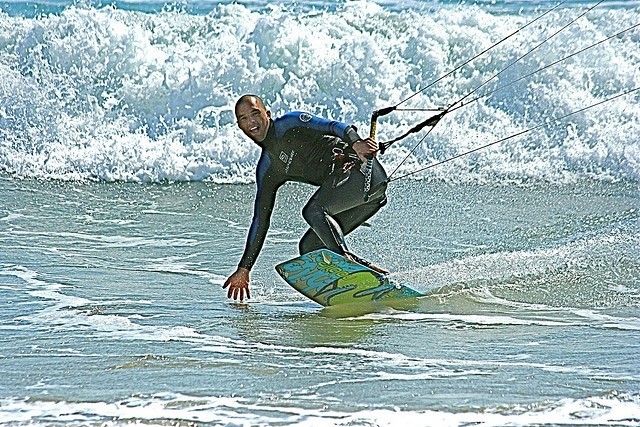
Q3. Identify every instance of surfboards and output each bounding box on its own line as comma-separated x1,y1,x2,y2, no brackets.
274,249,426,306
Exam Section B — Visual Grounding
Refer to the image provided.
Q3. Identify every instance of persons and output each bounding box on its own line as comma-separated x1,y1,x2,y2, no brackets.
222,95,388,301
